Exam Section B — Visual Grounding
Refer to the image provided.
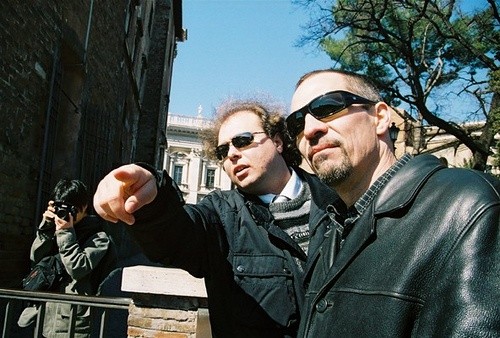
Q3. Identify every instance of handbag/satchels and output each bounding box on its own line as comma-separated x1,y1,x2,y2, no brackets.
24,255,72,293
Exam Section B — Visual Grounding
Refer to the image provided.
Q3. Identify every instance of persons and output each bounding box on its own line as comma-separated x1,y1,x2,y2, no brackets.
94,69,500,338
16,179,125,337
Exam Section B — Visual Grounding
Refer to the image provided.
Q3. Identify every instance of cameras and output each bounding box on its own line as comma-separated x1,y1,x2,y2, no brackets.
52,200,78,222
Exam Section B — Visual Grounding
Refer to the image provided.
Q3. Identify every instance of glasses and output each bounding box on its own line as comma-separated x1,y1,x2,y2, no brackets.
286,90,376,140
215,131,264,161
